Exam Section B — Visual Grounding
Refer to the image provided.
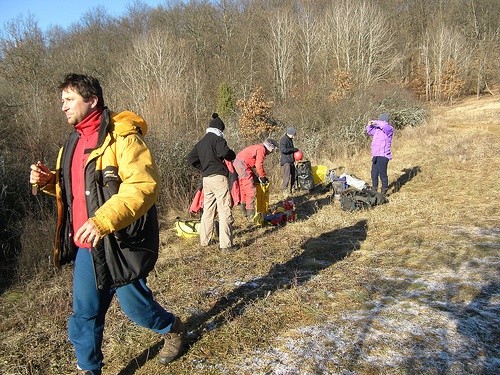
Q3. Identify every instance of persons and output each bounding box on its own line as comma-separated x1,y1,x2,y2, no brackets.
29,74,186,375
233,139,279,220
186,113,240,254
366,114,395,197
281,128,299,194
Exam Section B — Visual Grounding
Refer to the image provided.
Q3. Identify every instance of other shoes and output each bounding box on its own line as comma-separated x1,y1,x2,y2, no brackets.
222,244,240,254
203,239,216,247
75,369,102,375
381,189,388,196
157,316,187,365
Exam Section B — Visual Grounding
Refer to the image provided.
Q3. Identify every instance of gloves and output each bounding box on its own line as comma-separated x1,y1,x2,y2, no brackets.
260,176,268,184
294,148,298,152
253,175,260,185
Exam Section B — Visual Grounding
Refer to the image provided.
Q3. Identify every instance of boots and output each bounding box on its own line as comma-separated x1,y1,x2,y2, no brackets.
241,202,248,217
247,212,254,222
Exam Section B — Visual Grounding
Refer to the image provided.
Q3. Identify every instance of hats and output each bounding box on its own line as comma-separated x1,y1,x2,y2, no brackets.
287,127,296,136
378,112,389,121
209,113,225,130
263,138,278,153
